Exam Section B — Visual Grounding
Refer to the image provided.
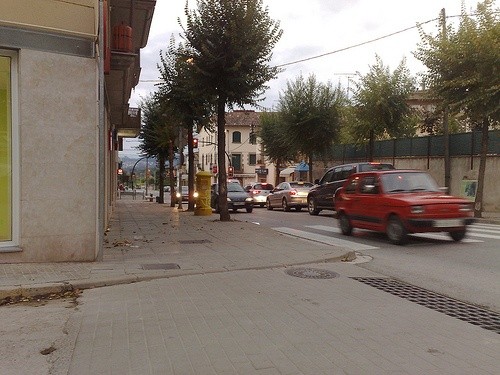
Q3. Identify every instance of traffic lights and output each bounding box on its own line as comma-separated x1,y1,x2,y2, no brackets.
228,166,234,179
193,138,199,148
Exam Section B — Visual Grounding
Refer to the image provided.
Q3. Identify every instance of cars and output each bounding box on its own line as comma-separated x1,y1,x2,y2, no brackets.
334,169,477,244
249,183,275,207
174,186,189,204
266,180,316,212
245,184,253,193
210,181,253,213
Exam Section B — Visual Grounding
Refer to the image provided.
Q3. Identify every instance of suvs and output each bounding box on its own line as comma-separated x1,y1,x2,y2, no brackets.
307,162,402,216
